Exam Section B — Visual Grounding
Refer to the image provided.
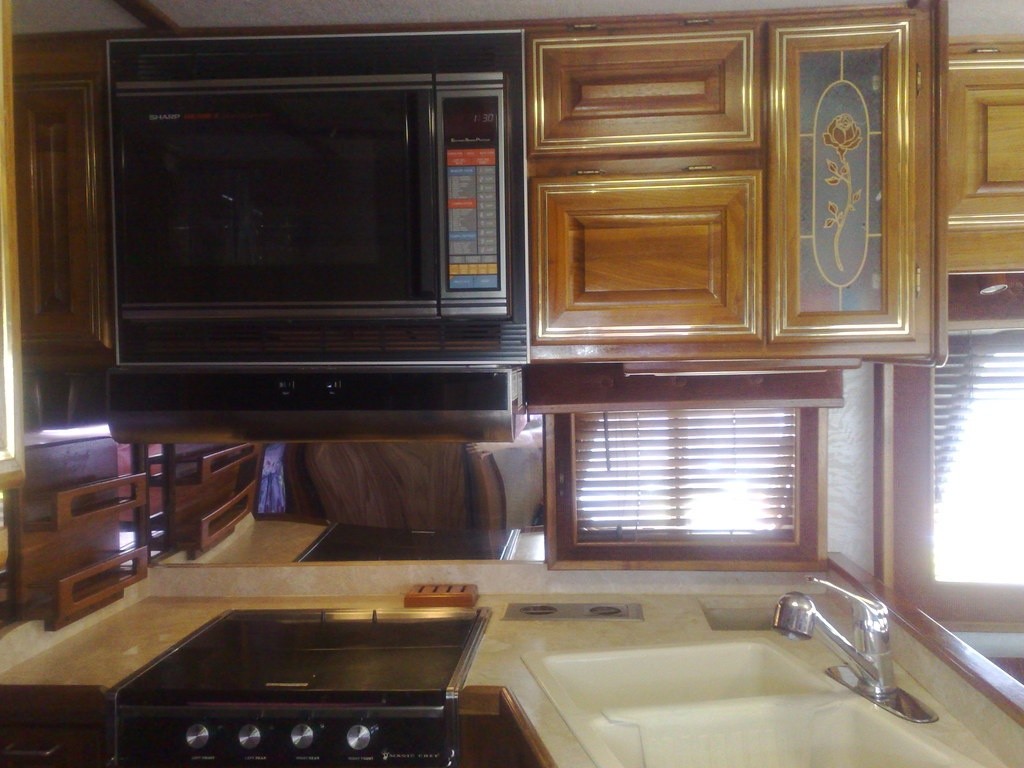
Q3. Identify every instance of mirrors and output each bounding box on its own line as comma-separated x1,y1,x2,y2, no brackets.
930,332,1024,686
118,415,546,563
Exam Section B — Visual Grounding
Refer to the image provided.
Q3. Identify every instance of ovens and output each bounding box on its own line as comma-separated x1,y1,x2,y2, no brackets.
104,27,532,369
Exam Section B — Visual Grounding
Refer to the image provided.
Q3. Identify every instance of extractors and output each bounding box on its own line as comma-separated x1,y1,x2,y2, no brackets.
102,365,530,445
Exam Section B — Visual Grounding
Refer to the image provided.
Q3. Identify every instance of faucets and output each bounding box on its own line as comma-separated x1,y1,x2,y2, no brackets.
770,574,940,723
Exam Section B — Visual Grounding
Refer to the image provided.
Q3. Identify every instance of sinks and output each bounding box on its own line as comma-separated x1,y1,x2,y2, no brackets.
520,636,857,711
554,701,1024,768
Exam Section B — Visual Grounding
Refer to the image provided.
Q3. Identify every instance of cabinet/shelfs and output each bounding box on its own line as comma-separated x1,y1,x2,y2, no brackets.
949,35,1024,274
149,443,264,560
13,33,115,372
527,6,949,366
16,438,148,632
0,685,110,768
